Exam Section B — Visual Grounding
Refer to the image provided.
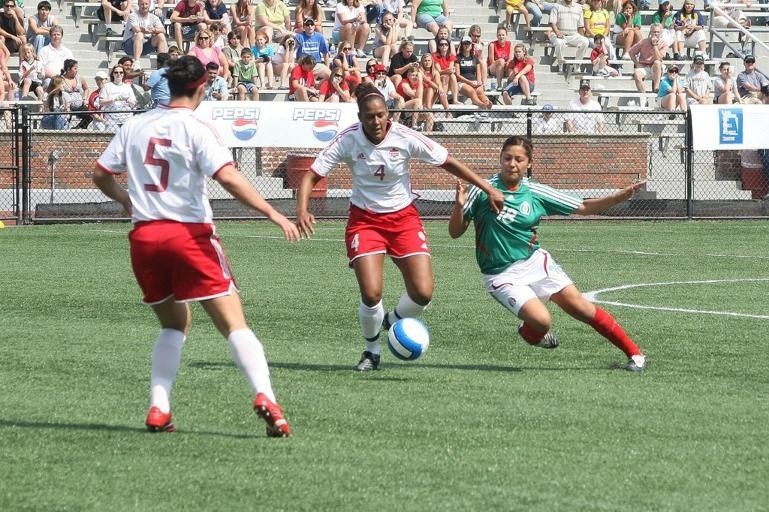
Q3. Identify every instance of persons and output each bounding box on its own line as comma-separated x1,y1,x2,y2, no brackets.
446,132,648,371
91,54,303,440
2,0,769,135
295,83,505,371
756,85,769,202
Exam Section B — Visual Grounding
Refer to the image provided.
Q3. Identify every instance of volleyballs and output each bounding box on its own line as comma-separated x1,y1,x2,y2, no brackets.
387,316,429,361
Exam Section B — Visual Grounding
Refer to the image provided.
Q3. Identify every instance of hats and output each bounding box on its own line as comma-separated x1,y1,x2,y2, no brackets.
540,104,553,110
461,1,757,73
577,79,592,89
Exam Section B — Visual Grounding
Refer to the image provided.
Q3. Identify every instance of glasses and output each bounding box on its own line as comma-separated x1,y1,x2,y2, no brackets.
1,1,452,82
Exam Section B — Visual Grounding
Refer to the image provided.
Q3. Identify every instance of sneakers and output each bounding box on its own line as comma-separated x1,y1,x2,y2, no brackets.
144,407,177,434
252,394,290,439
384,311,396,332
354,350,380,372
625,355,647,374
518,322,558,351
